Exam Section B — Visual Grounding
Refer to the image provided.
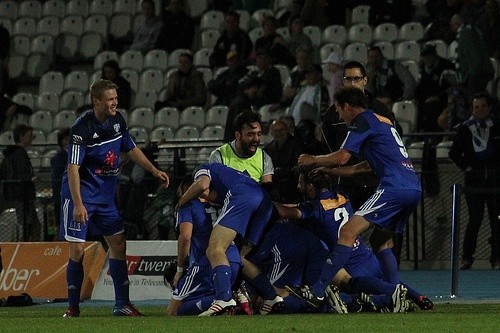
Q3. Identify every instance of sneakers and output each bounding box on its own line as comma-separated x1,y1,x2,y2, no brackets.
326,284,348,314
197,298,237,317
400,299,418,311
63,306,81,318
258,295,284,315
113,303,146,317
460,261,470,270
285,283,324,308
232,282,254,315
379,283,407,314
356,291,377,313
418,296,433,310
491,262,499,269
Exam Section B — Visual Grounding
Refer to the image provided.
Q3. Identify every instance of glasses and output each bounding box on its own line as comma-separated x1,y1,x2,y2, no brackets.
343,76,364,83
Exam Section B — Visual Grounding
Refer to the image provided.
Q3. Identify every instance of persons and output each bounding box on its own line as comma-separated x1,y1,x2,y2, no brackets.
59,78,170,319
0,0,500,316
296,87,423,313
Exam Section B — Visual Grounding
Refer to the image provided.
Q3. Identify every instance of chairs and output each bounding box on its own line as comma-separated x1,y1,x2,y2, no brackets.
0,0,500,243
